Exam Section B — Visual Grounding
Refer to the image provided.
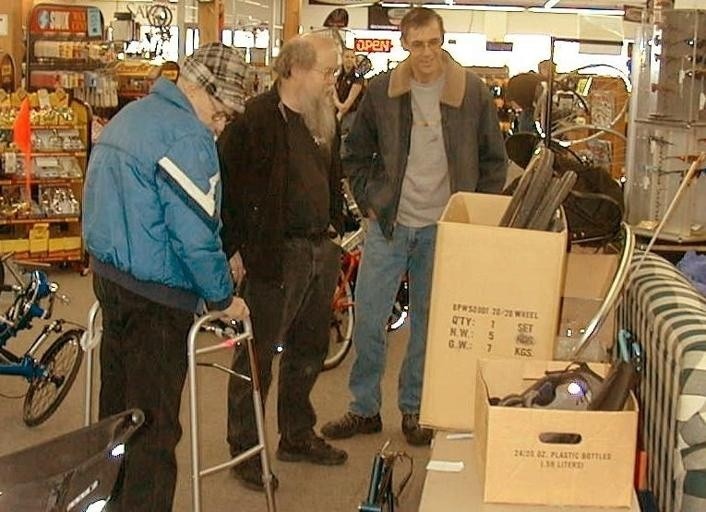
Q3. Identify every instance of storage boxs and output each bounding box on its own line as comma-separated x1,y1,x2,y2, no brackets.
555,243,621,364
419,193,568,434
473,355,640,505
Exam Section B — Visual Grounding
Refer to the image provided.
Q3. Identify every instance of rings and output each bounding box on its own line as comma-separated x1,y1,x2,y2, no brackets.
231,271,238,274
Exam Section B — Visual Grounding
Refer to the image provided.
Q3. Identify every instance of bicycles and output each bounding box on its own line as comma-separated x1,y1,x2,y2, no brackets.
2,262,85,429
318,242,409,370
140,6,174,60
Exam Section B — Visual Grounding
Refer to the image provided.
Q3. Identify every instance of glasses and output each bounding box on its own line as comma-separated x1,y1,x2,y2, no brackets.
208,94,235,123
312,64,338,80
403,35,445,51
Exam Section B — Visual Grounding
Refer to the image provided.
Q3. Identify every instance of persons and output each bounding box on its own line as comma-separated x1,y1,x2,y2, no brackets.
320,6,508,449
81,41,260,511
215,35,347,492
507,73,586,136
332,49,366,136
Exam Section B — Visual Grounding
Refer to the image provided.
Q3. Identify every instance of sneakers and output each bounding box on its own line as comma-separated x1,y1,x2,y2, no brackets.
276,432,347,464
321,413,383,440
403,415,427,444
230,452,279,491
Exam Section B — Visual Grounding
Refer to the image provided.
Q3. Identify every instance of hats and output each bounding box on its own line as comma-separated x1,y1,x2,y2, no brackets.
180,41,259,114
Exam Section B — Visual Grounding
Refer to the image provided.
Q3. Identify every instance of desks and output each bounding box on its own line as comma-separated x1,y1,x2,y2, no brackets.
417,430,644,512
